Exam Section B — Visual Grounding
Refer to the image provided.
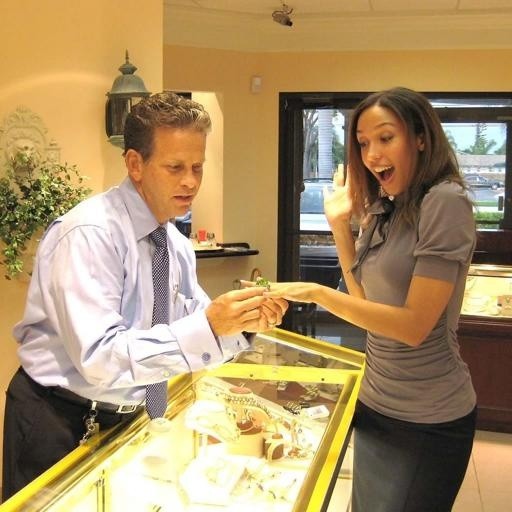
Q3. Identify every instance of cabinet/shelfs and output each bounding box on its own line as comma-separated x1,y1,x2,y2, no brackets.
0,319,370,512
455,264,512,437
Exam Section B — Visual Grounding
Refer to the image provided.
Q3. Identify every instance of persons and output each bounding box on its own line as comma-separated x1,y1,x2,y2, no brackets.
239,85,477,512
3,90,291,502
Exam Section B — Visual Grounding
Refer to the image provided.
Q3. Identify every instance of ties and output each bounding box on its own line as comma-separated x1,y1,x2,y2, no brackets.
146,226,168,419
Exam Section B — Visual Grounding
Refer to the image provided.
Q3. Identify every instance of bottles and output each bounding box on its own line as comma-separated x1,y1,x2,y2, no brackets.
176,211,190,238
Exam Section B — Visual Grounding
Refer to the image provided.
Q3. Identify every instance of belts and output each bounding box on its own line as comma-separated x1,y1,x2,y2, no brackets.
51,385,137,415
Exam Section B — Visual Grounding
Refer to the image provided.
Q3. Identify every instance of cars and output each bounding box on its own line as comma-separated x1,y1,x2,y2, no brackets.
301,178,334,231
465,175,505,190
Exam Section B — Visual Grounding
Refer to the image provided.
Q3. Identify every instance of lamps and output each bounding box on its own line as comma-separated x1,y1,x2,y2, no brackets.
103,50,152,151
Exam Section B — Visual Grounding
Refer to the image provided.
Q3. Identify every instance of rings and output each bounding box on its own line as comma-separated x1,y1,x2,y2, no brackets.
256,277,271,291
333,189,337,192
267,320,279,327
